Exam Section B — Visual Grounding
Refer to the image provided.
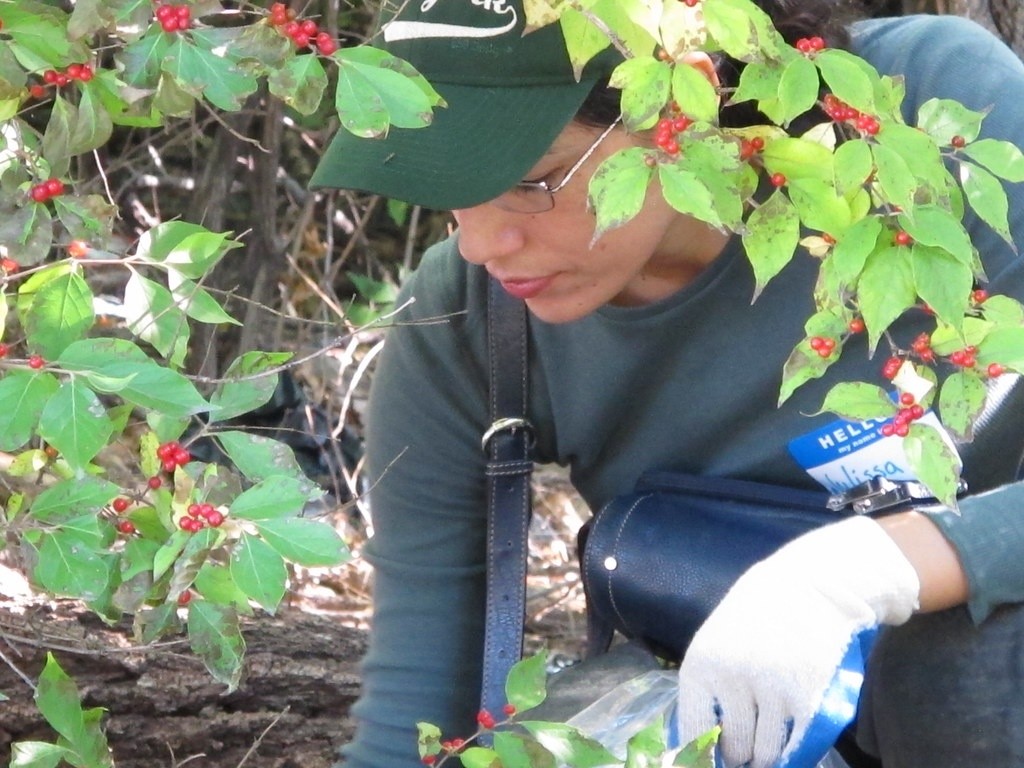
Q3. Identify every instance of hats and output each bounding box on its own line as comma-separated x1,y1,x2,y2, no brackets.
309,0,661,210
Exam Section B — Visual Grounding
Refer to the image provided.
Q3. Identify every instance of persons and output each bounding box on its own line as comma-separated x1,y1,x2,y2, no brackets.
306,0,1024,766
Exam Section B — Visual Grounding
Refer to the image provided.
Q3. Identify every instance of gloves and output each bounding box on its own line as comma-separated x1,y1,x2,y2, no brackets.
671,519,922,768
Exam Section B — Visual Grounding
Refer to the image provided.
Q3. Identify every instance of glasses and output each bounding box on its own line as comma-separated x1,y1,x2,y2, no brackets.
495,110,630,213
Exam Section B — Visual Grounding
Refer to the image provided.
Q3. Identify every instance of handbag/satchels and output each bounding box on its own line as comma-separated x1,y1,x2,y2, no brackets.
577,477,875,676
